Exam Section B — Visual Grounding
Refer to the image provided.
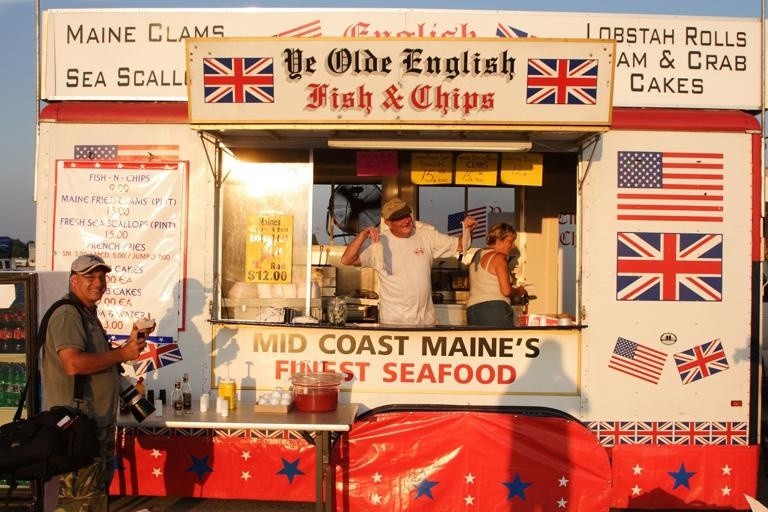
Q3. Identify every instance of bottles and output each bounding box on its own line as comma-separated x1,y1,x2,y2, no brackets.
0,363,28,407
0,311,25,353
136,374,192,416
200,381,237,417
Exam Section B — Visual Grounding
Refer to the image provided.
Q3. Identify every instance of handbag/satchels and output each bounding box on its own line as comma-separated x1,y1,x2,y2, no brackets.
0,406,102,480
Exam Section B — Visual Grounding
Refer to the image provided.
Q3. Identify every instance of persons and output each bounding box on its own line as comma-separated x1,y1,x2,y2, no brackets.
31,252,159,512
338,196,478,328
460,222,528,329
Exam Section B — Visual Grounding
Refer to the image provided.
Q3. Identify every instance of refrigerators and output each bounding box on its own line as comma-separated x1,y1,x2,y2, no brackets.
0,270,71,424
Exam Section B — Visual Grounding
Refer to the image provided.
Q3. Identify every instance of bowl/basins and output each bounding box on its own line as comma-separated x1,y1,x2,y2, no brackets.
291,372,342,413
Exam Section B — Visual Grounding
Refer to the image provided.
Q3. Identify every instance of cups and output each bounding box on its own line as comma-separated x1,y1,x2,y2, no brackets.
331,297,348,325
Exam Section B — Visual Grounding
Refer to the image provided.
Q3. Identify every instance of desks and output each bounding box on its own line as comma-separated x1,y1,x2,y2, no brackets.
114,402,359,511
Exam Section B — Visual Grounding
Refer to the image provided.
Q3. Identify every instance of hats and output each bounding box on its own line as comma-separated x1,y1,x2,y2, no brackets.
382,198,412,220
71,254,112,274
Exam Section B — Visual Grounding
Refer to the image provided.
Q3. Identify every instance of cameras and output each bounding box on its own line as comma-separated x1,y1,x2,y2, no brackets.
117,362,156,423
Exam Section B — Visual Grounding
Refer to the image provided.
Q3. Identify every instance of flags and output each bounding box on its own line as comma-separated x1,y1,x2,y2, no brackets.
527,58,599,106
202,56,275,105
617,150,726,224
124,342,182,377
615,231,724,302
673,337,730,385
607,336,669,386
